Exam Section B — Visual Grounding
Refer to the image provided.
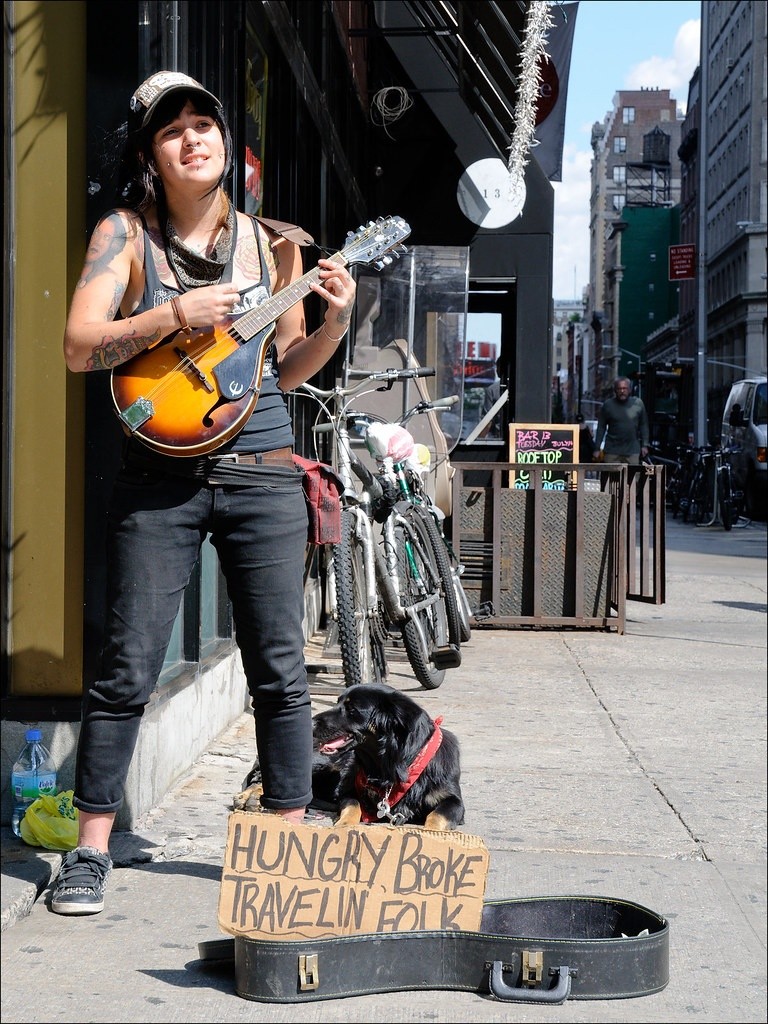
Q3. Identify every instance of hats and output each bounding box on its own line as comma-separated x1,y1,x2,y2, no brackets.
128,71,222,135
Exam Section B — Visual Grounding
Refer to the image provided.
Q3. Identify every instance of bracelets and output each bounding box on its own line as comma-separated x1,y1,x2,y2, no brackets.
172,296,194,333
322,321,350,342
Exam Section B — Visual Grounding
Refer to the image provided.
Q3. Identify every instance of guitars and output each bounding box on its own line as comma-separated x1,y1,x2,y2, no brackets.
102,206,421,467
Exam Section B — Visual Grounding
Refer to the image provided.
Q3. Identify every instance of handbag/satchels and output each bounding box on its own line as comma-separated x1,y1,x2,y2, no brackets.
20,789,79,851
291,453,344,546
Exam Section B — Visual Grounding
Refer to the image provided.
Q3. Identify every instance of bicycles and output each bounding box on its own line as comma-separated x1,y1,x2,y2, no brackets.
656,429,749,531
272,365,495,706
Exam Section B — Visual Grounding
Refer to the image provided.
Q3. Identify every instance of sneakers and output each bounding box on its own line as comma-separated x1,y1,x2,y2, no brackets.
52,846,112,913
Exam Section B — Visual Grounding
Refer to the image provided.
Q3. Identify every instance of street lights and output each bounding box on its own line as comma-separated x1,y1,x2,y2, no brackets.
599,344,641,402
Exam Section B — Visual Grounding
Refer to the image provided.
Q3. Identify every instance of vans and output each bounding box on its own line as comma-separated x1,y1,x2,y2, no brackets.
720,377,768,477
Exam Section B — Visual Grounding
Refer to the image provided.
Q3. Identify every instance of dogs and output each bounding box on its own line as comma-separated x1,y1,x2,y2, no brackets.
311,684,466,833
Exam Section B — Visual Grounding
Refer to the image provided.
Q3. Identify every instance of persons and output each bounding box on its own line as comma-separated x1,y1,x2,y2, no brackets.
574,413,594,463
478,358,509,438
592,376,650,504
46,71,358,912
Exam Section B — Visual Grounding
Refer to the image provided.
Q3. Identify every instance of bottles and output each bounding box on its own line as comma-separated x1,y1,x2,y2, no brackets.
12,729,56,837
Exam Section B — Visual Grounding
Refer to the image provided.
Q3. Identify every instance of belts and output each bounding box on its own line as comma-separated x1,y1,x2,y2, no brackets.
207,447,295,484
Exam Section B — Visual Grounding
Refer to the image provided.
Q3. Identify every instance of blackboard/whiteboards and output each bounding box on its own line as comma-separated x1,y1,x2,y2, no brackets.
509,423,580,491
